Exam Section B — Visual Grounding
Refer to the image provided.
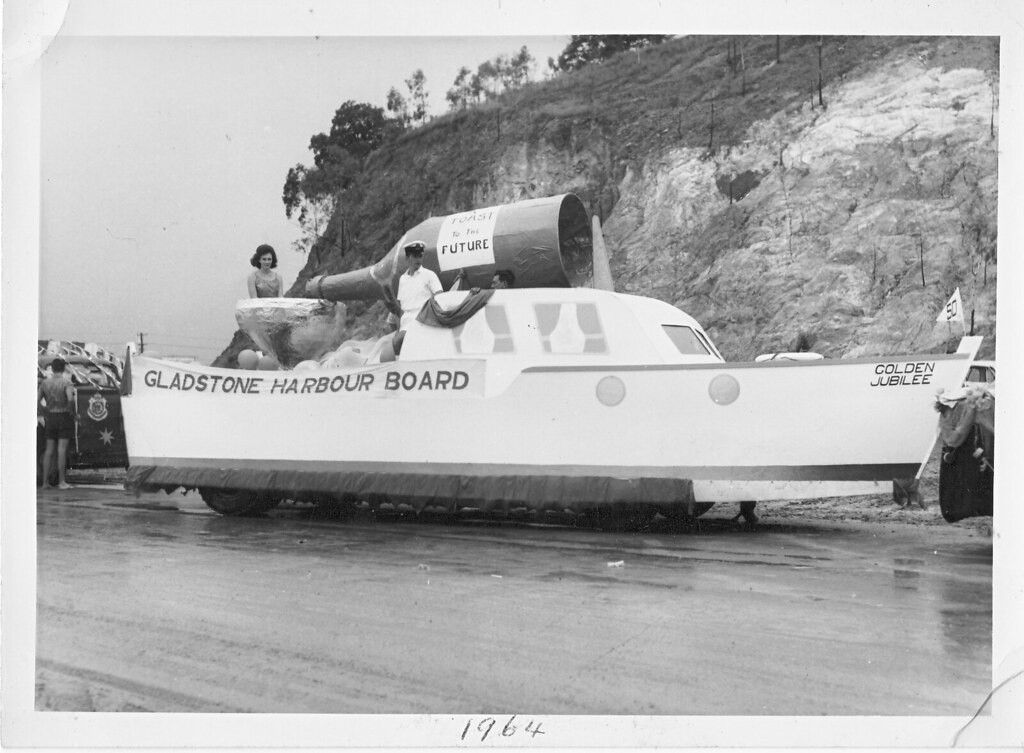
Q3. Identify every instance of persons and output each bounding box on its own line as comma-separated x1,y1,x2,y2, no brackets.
37,359,81,490
397,240,443,331
491,269,515,289
247,244,284,298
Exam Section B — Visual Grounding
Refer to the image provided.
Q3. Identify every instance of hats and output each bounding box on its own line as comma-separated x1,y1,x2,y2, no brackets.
403,240,426,251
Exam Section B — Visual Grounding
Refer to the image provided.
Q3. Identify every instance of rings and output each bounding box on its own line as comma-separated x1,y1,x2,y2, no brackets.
79,416,80,417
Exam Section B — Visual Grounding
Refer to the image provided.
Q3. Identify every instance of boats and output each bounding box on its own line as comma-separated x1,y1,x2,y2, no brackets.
118,287,984,524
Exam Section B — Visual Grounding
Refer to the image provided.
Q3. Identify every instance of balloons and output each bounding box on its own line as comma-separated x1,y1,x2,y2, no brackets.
238,345,396,373
